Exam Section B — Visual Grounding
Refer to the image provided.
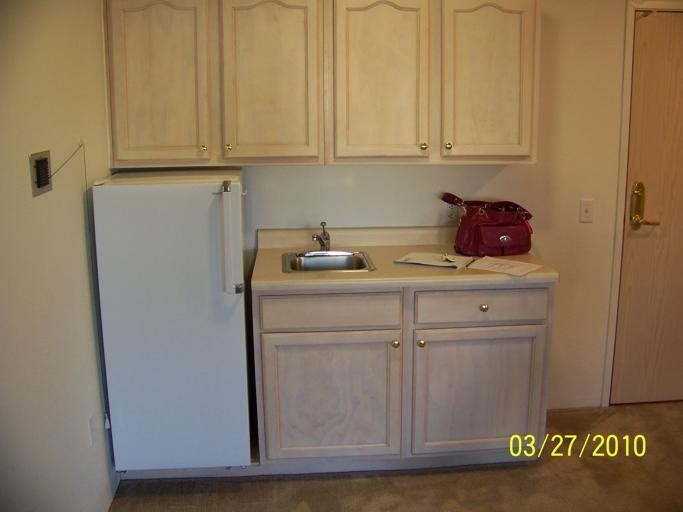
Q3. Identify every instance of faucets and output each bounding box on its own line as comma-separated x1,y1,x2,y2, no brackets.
311,219,331,251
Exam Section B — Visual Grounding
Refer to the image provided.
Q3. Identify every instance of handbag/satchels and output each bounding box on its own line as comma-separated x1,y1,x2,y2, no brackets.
441,192,533,257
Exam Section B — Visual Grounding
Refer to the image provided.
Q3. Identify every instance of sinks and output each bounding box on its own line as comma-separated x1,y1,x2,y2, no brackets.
280,246,376,274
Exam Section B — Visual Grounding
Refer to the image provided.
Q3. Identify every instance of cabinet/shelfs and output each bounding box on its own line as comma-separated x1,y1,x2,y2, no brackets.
258,292,550,461
333,0,537,158
105,3,323,167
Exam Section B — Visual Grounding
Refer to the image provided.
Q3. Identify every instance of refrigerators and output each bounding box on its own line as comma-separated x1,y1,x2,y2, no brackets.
91,171,251,471
